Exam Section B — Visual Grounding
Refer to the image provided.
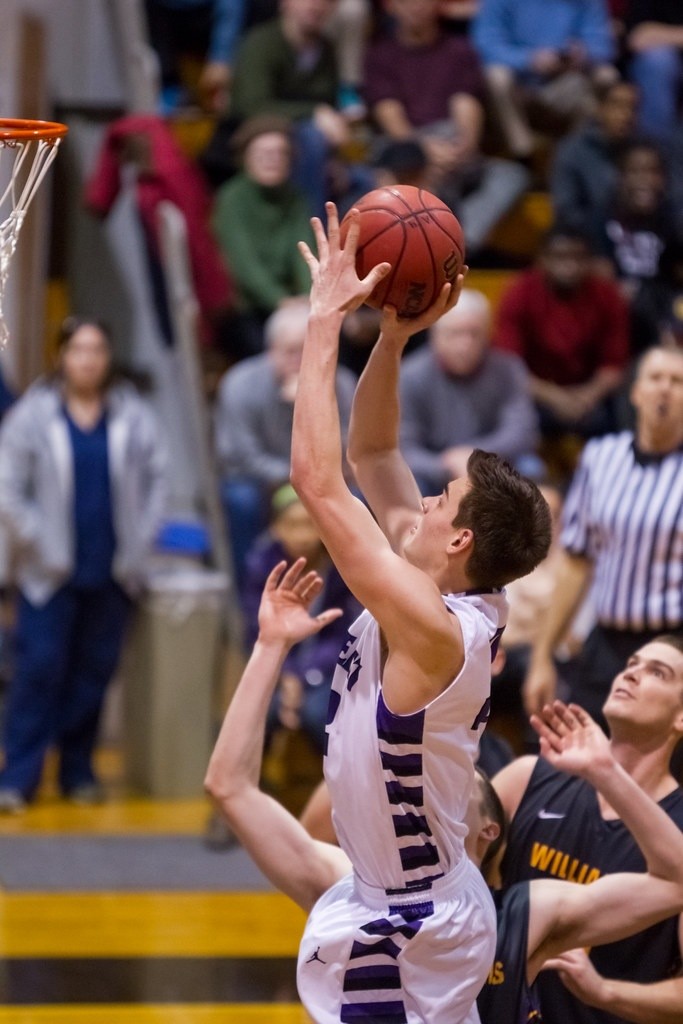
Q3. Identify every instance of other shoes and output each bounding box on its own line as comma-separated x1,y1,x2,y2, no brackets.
64,784,105,806
0,788,28,815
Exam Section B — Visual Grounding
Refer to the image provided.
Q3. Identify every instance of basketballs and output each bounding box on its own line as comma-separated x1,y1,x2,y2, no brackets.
337,183,466,317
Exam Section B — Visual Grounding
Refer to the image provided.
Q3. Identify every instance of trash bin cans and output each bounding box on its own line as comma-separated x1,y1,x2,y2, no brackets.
115,553,231,801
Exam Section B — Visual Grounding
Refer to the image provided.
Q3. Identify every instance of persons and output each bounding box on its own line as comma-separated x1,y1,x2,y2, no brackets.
0,313,170,815
289,193,556,1024
177,2,683,1024
